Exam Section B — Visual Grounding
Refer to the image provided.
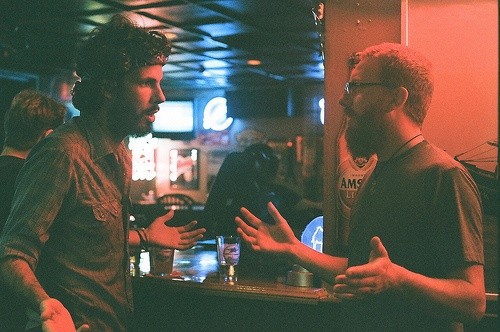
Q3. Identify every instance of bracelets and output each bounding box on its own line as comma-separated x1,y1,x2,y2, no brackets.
134,229,148,250
141,229,150,252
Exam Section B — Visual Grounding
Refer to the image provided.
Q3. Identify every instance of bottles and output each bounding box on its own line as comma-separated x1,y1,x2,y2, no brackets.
139,249,151,277
129,256,136,277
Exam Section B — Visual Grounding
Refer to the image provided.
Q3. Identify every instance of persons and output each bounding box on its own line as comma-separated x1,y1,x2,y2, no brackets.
0,89,69,230
234,42,486,332
0,14,207,332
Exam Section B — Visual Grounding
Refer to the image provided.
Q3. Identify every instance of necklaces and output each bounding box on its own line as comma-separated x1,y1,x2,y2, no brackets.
371,134,422,192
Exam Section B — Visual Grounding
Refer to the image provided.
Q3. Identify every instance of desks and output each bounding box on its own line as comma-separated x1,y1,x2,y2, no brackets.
129,239,329,332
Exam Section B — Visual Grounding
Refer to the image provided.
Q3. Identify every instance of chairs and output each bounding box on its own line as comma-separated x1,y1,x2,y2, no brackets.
157,194,194,227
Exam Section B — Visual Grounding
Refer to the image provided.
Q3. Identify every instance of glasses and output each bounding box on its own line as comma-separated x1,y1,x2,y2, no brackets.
345,82,387,95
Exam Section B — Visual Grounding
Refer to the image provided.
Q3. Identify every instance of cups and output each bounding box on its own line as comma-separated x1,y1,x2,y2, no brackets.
215,235,240,287
150,248,174,276
